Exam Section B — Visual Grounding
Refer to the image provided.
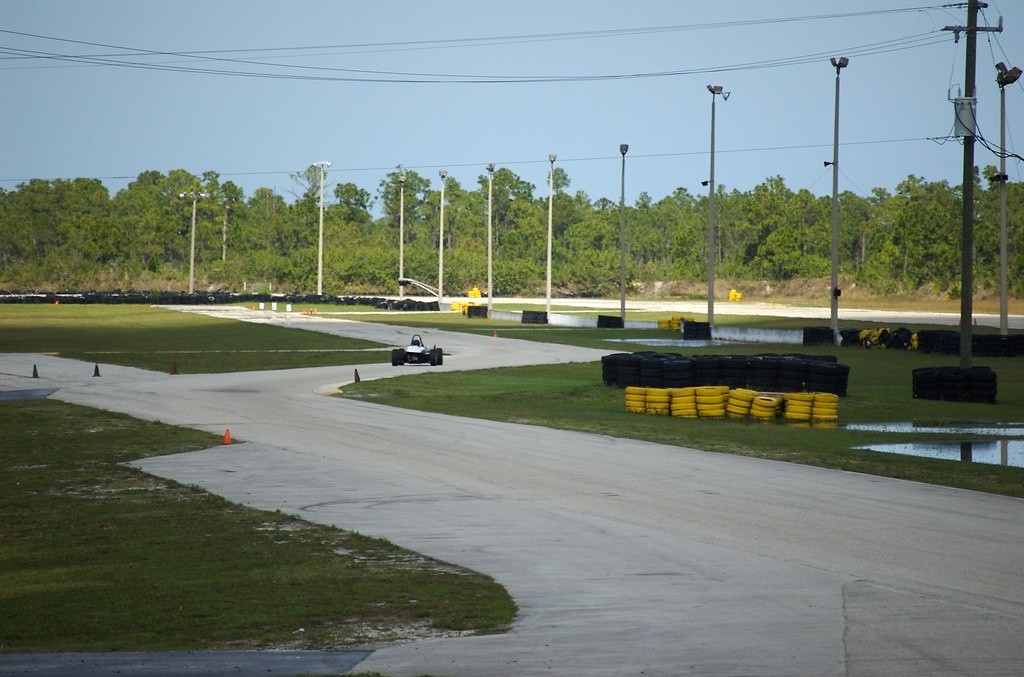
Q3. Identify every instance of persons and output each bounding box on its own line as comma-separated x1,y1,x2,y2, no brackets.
407,340,425,353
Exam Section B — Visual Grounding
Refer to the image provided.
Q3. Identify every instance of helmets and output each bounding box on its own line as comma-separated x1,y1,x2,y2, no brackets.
413,340,419,346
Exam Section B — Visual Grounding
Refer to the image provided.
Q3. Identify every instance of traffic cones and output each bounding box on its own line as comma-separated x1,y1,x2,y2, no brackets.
222,429,232,444
31,364,40,378
493,331,497,337
170,359,179,374
354,367,360,383
93,363,101,377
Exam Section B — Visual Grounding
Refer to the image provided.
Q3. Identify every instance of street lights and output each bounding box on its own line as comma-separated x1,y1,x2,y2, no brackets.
486,163,496,308
990,61,1023,334
701,85,731,325
399,176,404,299
620,144,628,321
437,170,447,304
824,57,849,330
180,192,206,295
547,155,557,312
313,162,331,295
